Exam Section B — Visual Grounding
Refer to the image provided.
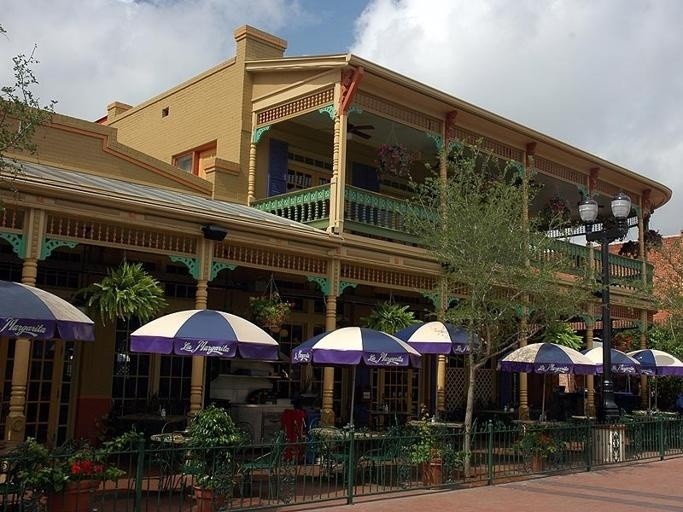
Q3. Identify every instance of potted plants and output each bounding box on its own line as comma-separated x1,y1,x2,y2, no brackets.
247,273,295,328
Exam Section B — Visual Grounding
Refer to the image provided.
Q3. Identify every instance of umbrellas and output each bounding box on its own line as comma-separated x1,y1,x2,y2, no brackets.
0,279,96,342
130,309,280,409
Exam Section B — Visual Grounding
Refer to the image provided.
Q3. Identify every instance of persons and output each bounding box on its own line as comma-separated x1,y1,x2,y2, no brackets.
279,398,309,463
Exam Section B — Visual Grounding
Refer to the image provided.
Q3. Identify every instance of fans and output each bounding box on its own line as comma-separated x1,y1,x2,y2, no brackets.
347,113,375,141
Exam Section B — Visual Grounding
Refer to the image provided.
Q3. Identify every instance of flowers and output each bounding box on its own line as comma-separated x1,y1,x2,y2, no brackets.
543,183,571,229
376,121,416,181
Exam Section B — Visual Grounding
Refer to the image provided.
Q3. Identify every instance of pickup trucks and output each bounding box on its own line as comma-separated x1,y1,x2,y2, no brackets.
559,392,619,421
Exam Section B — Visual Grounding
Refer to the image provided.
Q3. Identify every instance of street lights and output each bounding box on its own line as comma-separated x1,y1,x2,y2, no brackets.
577,192,632,424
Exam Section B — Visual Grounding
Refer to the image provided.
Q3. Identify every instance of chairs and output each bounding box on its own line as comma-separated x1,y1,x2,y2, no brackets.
0,408,683,512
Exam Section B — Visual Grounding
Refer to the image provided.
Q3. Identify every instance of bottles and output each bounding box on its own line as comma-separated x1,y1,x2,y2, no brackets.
432,414,435,424
